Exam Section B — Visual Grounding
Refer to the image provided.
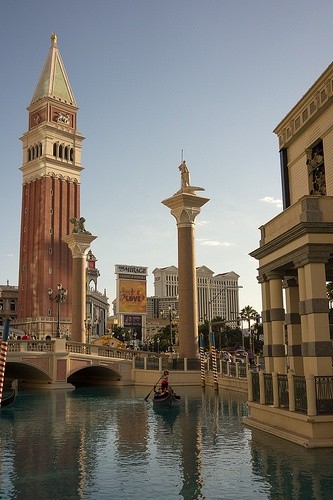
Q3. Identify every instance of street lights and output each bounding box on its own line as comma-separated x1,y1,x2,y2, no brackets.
47,283,68,339
84,316,97,354
145,337,151,352
230,311,244,351
209,286,243,351
157,338,160,354
160,305,177,352
131,332,137,350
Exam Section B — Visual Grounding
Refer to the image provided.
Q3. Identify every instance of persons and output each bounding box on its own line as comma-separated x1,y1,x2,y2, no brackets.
105,341,137,350
9,332,29,340
222,352,231,362
161,370,169,389
154,386,174,399
247,349,256,366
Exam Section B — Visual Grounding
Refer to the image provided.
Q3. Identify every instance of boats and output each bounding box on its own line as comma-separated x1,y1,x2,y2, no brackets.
152,384,181,408
0,388,15,410
